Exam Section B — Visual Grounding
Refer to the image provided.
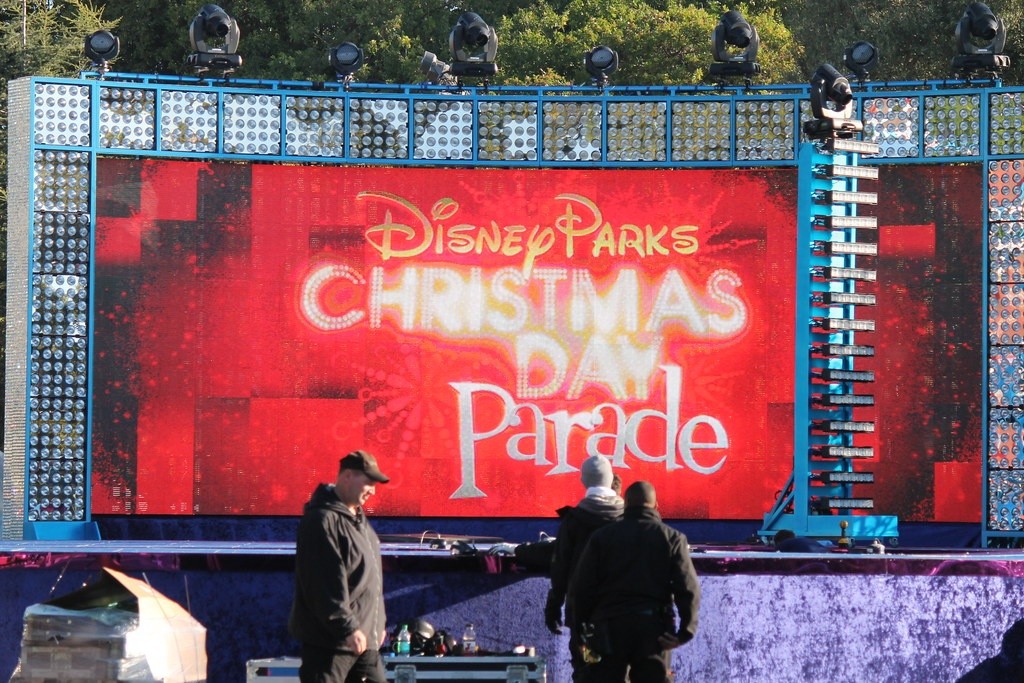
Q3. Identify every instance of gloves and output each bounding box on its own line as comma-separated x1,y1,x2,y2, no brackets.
546,604,563,635
657,632,688,650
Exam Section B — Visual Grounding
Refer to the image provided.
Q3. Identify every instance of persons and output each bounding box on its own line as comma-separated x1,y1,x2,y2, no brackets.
544,454,625,683
611,472,623,497
293,450,391,683
567,480,701,683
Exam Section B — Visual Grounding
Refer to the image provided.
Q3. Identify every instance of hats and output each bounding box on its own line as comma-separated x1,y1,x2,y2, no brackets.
339,450,390,484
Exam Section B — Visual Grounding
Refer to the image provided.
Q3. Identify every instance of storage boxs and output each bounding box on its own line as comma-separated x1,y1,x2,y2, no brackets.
22,611,208,683
246,656,302,683
381,656,548,683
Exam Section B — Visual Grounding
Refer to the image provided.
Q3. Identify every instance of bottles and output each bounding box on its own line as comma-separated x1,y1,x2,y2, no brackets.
397,625,411,657
463,624,476,655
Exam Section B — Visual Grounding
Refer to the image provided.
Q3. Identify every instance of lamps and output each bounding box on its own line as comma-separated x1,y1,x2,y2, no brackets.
188,4,242,78
707,11,762,92
449,12,498,92
802,64,863,140
331,42,364,87
85,29,121,81
843,41,879,86
950,2,1011,85
583,47,620,90
